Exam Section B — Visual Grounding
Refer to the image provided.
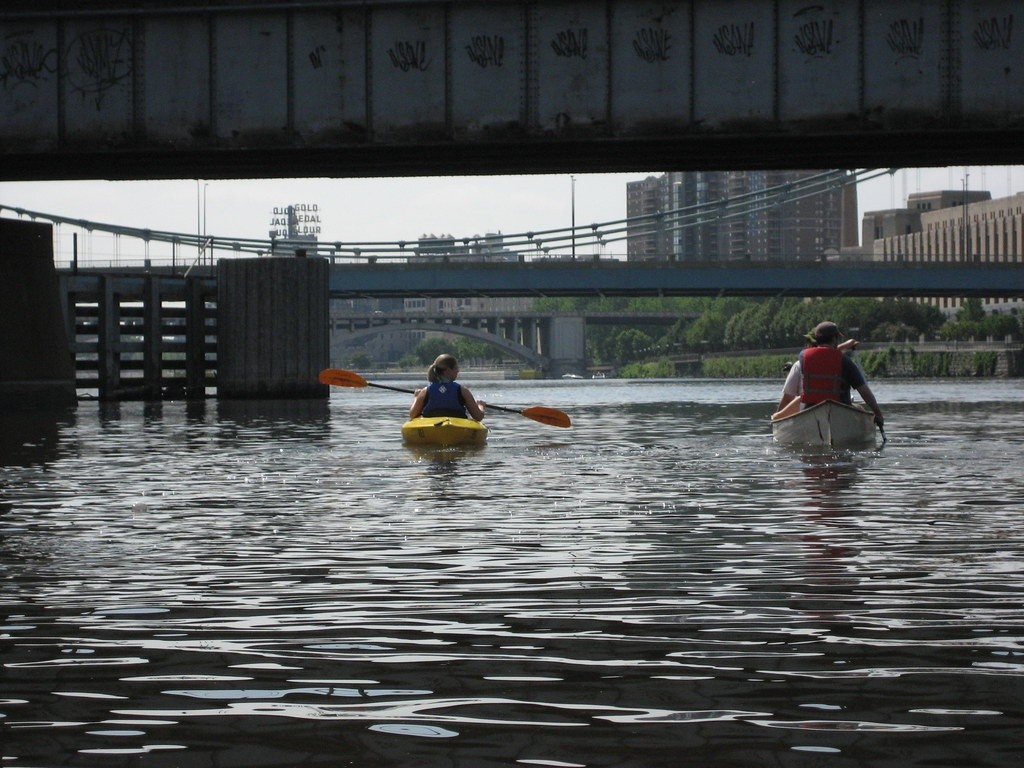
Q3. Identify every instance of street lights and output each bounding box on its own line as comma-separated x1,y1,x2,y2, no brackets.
960,174,972,261
569,173,576,262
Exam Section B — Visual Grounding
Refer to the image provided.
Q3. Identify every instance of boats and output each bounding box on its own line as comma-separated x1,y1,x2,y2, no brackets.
399,414,489,447
771,395,878,450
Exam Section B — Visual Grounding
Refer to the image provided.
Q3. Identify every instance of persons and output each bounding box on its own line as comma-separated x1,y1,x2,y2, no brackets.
772,322,884,427
410,354,486,422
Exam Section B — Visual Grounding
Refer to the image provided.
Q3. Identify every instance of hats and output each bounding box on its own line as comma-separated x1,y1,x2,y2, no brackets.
803,327,817,343
816,321,844,338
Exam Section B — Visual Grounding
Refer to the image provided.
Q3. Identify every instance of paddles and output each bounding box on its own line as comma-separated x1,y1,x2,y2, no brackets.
319,369,571,429
876,424,890,441
